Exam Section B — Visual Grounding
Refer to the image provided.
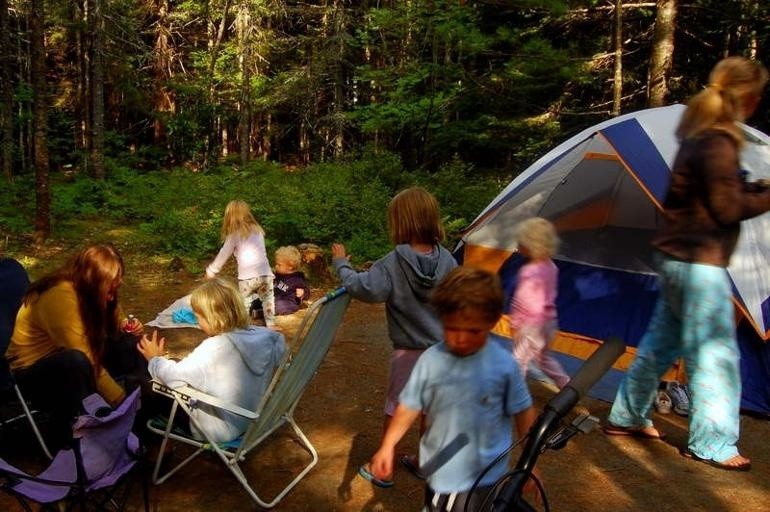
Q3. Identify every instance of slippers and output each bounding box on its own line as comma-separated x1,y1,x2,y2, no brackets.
684,450,750,471
401,458,425,479
359,464,395,488
605,425,668,439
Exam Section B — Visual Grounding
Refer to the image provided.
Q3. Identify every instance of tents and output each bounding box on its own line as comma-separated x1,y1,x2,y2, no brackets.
451,101,769,425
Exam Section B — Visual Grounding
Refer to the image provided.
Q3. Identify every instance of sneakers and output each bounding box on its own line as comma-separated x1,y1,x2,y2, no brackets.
655,391,672,414
666,381,689,415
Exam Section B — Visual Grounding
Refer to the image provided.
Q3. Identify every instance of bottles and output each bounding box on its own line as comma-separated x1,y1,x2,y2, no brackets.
124,314,134,333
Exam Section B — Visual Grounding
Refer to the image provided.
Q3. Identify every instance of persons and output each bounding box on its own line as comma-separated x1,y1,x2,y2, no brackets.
330,187,460,489
270,245,310,313
601,57,769,475
136,278,288,450
509,216,571,407
370,264,538,510
7,240,145,451
203,198,277,331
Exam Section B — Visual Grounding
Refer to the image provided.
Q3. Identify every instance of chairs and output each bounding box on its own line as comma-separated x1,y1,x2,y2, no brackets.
0,254,56,460
147,285,352,509
0,382,151,511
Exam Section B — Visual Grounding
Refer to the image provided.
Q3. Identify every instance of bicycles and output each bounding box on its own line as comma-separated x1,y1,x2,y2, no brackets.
464,338,627,511
